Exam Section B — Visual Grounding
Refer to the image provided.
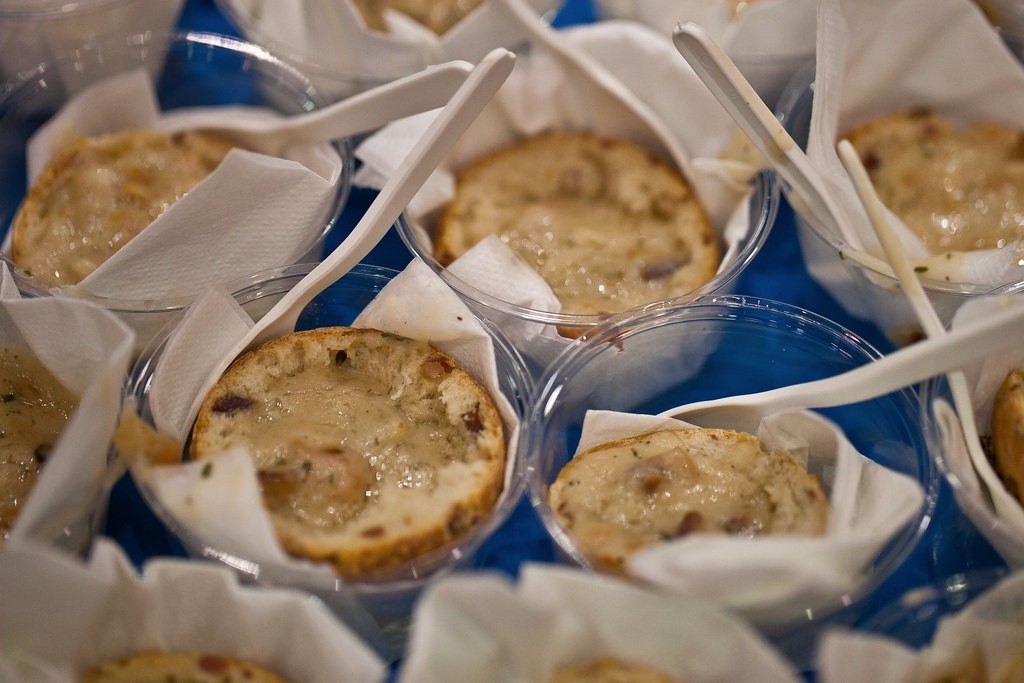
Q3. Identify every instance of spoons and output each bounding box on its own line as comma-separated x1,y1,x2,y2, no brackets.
152,58,471,155
654,307,1024,432
838,138,1022,540
171,44,518,462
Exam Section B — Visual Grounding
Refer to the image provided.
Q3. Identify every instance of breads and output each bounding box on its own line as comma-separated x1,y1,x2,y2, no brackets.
191,324,507,577
542,661,679,683
86,651,298,683
547,427,829,576
11,128,280,293
832,107,1024,265
989,366,1024,510
437,123,719,339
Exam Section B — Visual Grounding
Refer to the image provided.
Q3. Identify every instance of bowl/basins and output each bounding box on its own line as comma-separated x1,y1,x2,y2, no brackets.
920,277,1024,578
594,0,816,100
378,161,782,341
0,1,187,112
767,26,1024,322
215,1,569,116
821,573,1023,683
519,294,938,663
118,260,544,594
1,30,353,354
